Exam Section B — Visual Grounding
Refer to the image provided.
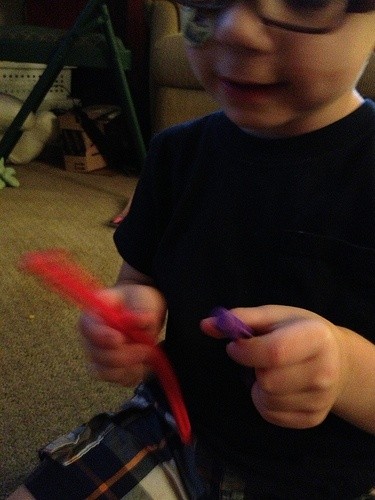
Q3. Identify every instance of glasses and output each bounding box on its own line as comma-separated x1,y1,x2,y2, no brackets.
176,1,375,34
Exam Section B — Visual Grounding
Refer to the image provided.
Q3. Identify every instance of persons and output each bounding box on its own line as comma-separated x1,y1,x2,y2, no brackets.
1,0,375,500
